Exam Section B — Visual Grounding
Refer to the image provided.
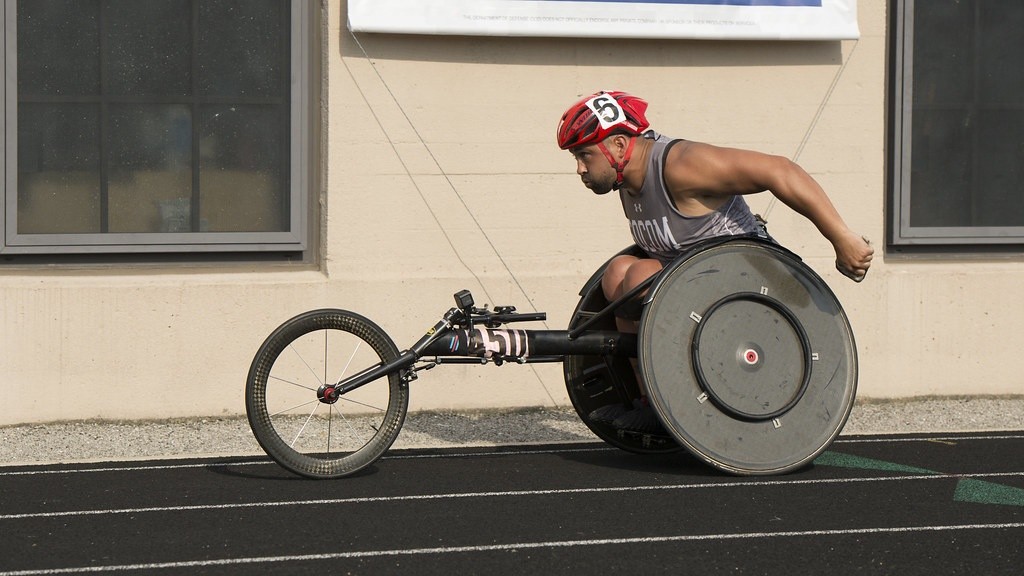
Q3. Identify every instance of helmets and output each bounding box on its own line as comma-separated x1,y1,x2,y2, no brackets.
557,90,650,150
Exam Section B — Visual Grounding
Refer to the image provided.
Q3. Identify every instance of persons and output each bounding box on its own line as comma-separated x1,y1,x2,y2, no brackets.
557,90,874,431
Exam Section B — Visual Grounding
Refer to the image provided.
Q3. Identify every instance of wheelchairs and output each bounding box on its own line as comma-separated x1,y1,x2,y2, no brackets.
245,213,860,478
